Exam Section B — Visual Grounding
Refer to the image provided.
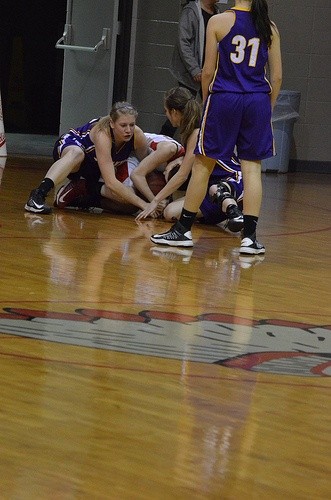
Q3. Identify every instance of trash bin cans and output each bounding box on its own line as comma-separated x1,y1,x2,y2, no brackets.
259,91,300,173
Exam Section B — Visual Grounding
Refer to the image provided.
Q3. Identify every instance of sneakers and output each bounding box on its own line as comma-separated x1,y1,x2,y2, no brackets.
239,233,266,254
24,191,52,214
149,221,194,247
53,179,85,208
226,206,244,233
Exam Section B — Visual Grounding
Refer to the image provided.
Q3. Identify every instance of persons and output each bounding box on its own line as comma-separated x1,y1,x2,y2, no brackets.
148,0,282,256
24,0,245,233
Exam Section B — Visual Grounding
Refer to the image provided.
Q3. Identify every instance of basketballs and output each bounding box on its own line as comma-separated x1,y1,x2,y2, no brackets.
135,173,174,209
